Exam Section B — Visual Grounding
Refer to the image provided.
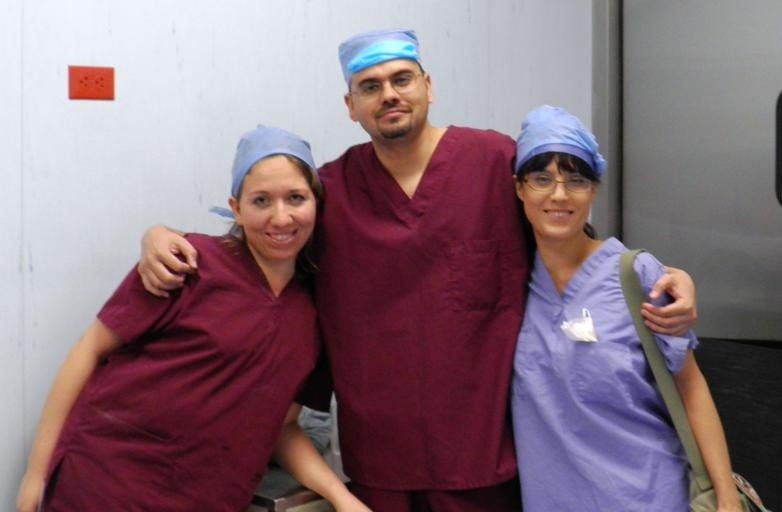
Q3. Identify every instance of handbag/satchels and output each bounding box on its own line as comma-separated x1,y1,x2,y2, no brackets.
689,472,756,512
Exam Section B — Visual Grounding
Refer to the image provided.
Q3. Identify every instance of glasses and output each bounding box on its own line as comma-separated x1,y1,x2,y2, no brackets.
524,170,591,193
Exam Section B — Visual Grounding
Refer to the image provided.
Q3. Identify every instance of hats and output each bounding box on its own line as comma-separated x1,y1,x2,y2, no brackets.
232,124,319,198
338,28,419,86
514,106,605,175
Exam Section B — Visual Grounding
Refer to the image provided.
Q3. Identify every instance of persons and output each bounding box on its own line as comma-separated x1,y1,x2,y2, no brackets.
137,28,696,512
512,106,744,512
16,125,375,512
